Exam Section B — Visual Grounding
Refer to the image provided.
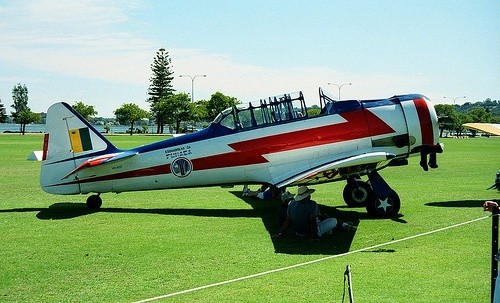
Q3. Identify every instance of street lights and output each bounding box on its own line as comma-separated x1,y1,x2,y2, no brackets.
179,74,207,131
328,82,353,101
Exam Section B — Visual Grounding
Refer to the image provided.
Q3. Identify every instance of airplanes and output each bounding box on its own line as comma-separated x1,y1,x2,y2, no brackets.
26,89,454,219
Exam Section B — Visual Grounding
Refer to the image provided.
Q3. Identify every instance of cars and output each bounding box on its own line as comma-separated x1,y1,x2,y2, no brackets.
126,128,139,133
